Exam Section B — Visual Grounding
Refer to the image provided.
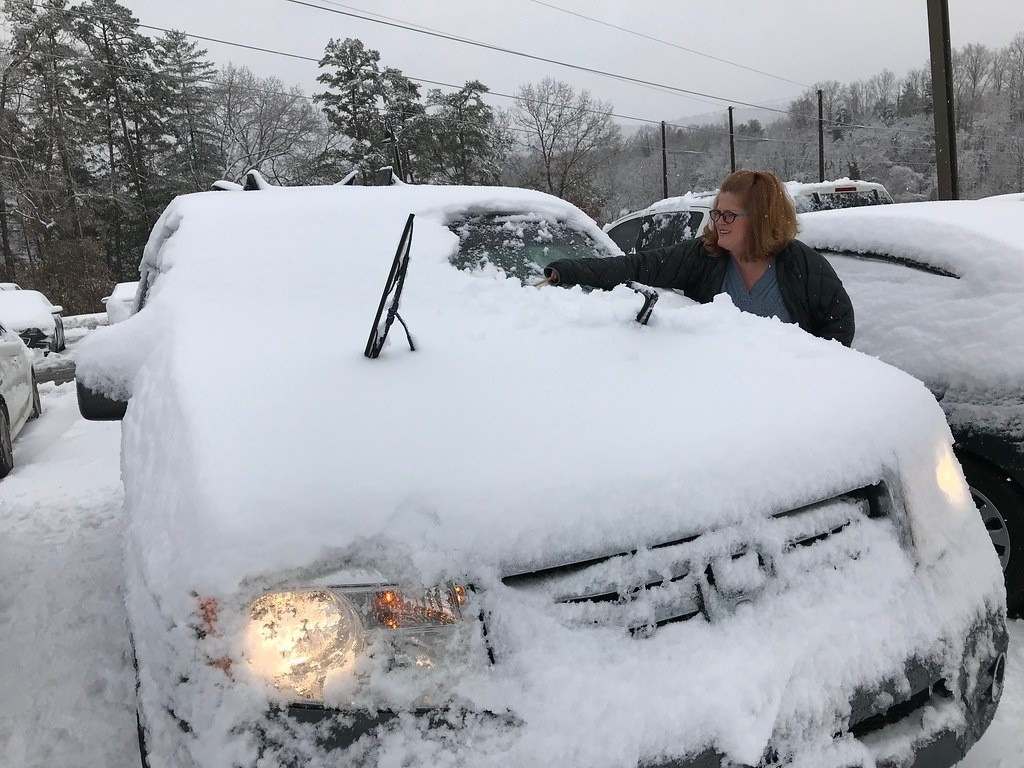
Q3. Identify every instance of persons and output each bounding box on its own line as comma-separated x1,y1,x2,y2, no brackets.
526,170,856,349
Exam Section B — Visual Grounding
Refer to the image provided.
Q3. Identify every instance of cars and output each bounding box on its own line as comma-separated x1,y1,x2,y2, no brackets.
0,322,41,475
603,183,898,254
101,282,140,325
0,289,66,354
75,185,1024,768
747,194,1024,621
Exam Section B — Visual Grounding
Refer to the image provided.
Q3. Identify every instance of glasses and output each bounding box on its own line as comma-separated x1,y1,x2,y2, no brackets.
709,209,751,224
